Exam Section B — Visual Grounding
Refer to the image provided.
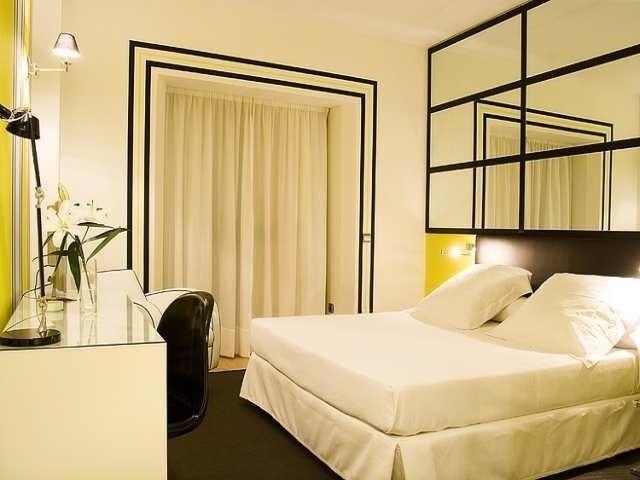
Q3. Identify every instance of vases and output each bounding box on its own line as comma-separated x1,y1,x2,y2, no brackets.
74,257,99,313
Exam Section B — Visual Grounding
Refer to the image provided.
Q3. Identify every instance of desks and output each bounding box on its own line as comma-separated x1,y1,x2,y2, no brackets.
0,269,168,480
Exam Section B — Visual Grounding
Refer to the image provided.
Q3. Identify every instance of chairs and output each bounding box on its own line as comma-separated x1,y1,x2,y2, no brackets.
35,263,221,439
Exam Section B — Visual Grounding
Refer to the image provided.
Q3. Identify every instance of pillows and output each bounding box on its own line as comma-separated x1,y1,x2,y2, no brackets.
409,264,640,370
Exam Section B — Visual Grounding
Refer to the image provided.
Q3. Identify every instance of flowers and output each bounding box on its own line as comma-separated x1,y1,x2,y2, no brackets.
33,180,131,314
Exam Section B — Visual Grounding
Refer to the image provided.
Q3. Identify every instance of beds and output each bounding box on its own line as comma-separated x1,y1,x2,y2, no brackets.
239,235,640,480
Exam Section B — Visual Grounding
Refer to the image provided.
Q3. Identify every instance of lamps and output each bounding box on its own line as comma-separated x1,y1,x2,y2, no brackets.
25,32,83,76
1,107,62,347
